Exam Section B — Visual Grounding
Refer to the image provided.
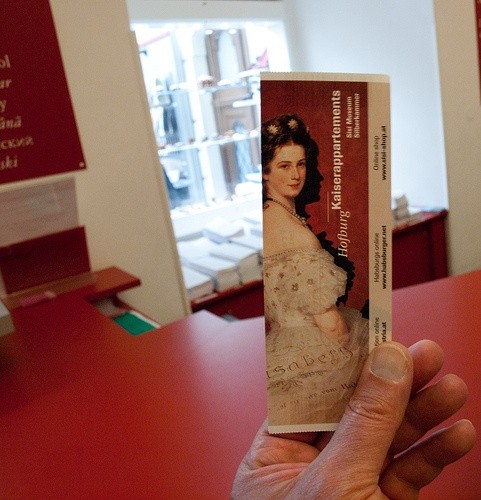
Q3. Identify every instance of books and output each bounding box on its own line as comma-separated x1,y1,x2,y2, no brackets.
175,235,219,264
181,267,214,302
392,192,411,220
208,242,261,284
189,255,240,291
172,207,262,238
228,233,262,262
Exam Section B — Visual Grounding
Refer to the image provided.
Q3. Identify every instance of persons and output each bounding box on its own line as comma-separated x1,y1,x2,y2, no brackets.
227,339,477,500
